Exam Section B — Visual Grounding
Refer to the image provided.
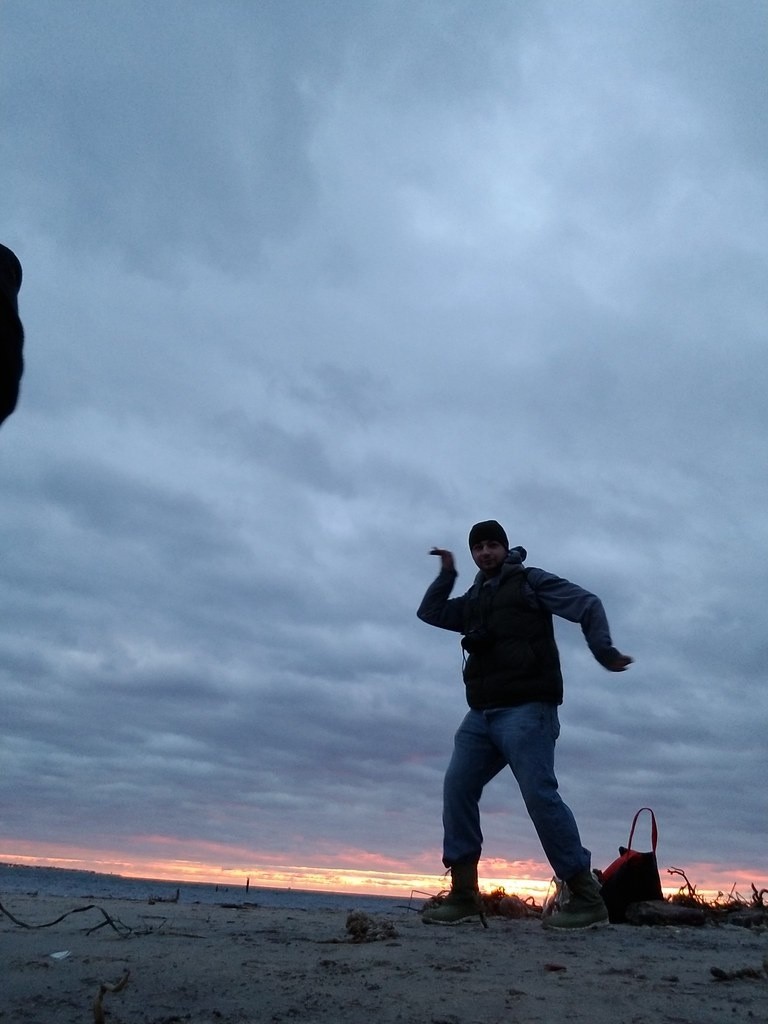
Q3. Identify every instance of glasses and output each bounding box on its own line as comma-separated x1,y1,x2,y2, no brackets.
473,543,500,551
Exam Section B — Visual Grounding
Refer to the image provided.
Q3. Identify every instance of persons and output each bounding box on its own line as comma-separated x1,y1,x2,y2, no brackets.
417,519,634,934
0,243,25,425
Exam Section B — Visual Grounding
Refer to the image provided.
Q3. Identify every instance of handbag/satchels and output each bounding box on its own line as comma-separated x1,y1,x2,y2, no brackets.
592,808,665,925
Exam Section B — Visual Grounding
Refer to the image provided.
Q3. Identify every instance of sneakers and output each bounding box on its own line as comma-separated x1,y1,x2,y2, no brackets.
540,872,610,931
422,886,490,928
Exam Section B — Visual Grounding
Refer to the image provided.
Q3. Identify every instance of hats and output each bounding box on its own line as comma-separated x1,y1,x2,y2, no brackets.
469,520,509,553
